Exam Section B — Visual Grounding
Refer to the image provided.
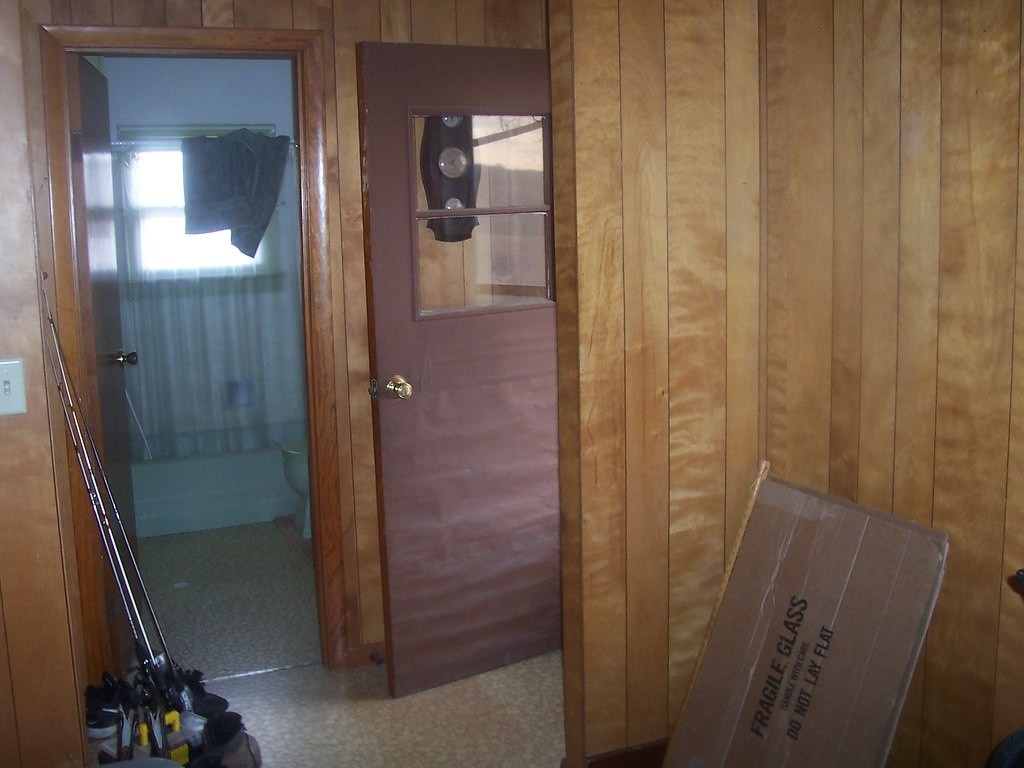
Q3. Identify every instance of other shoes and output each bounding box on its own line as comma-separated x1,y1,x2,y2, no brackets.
178,669,229,716
102,671,131,707
85,684,118,738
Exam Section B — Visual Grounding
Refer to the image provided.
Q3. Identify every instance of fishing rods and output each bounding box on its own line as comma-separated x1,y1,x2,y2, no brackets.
37,258,175,686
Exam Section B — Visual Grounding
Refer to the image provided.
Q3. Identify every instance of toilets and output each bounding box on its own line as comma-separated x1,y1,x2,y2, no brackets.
281,445,315,539
